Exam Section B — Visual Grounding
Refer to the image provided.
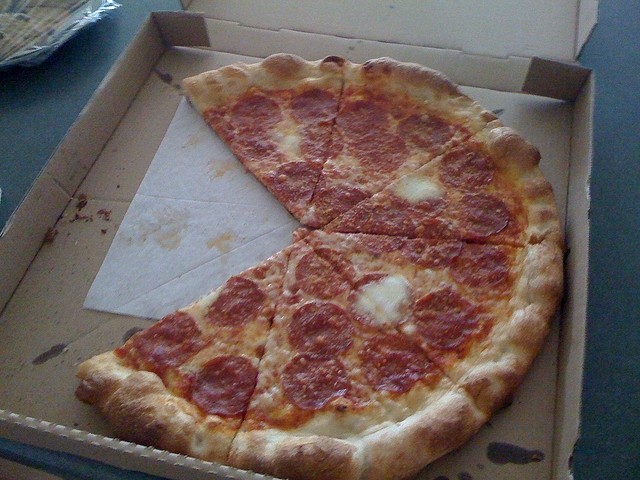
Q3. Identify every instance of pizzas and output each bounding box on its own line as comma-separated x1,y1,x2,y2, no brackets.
75,53,566,480
